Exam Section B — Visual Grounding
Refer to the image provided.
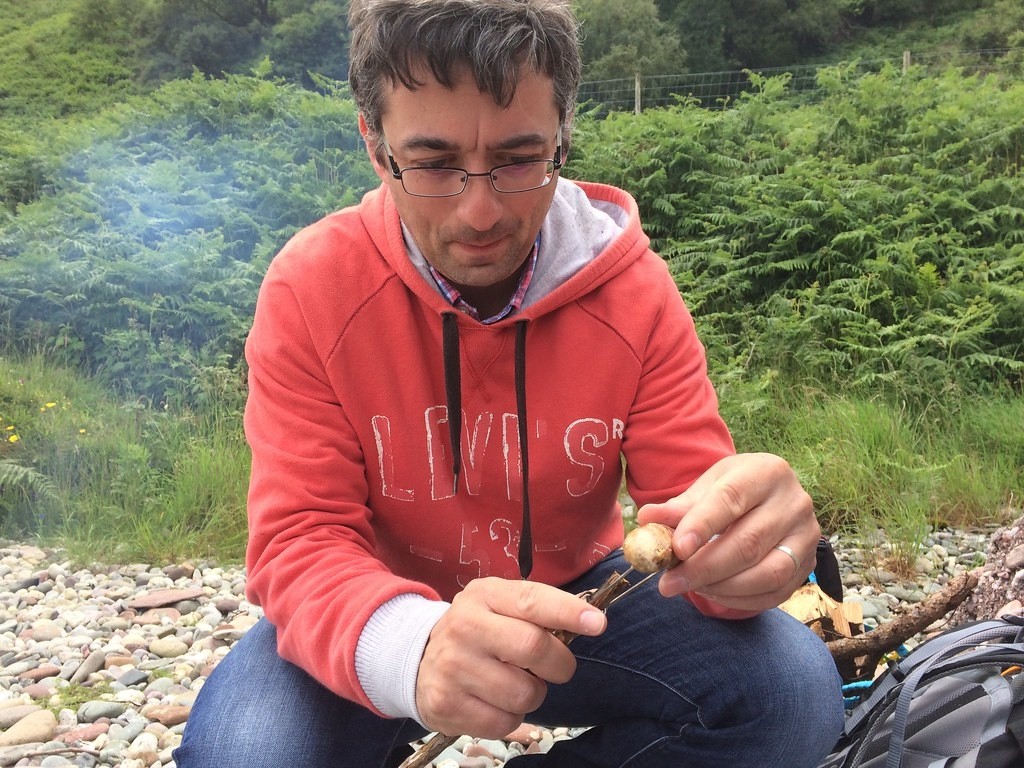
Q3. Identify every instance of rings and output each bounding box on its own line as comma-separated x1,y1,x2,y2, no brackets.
774,543,801,575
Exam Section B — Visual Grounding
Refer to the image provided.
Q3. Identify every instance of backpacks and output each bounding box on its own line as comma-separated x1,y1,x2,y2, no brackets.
819,612,1023,767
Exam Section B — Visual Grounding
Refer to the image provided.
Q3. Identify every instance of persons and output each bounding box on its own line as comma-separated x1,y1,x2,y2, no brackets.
171,0,846,768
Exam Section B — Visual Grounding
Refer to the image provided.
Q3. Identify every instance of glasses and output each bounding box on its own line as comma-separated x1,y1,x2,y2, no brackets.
381,128,563,198
